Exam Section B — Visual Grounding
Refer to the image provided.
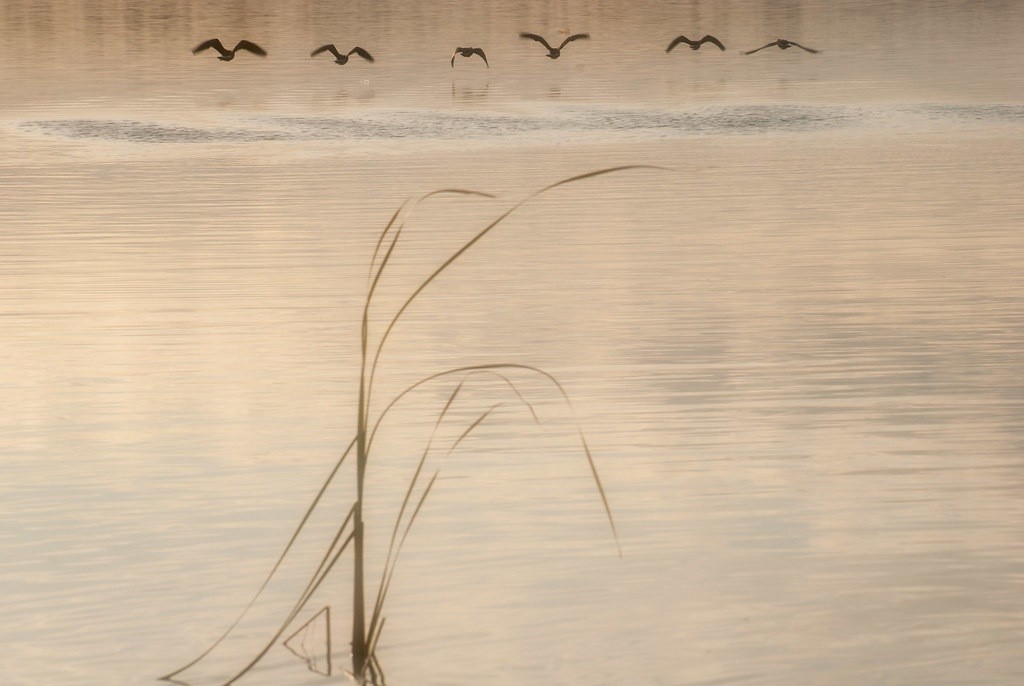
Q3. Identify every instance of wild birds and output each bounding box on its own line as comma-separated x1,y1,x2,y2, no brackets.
450,47,489,69
311,44,375,65
666,35,726,53
744,39,818,55
519,34,590,59
191,38,268,61
